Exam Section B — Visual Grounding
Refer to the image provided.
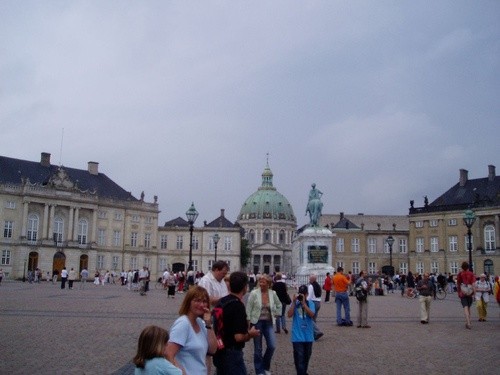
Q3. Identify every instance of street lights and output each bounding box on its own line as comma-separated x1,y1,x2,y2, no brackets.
213,233,220,261
462,210,476,268
185,202,199,271
386,235,394,278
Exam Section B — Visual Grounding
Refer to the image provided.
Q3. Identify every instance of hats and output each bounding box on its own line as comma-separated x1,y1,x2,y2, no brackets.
298,285,307,293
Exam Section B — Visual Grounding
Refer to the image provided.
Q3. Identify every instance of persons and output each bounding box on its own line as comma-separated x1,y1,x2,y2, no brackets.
333,267,353,327
416,272,433,324
457,262,477,329
165,286,218,375
485,272,500,304
474,273,491,322
0,268,4,282
429,272,457,296
94,267,151,295
198,260,228,375
160,269,204,297
288,285,315,375
306,275,324,341
355,270,371,328
28,266,89,289
134,326,185,375
305,184,323,216
366,270,423,298
210,272,260,375
324,272,337,304
246,273,282,375
346,271,354,296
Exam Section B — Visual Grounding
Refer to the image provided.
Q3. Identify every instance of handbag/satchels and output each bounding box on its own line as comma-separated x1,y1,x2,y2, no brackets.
461,283,474,295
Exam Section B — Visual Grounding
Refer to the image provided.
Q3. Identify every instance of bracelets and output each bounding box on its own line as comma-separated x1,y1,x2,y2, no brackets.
206,325,211,329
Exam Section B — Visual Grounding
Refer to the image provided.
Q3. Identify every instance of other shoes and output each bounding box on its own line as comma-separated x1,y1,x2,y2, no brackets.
275,330,281,333
478,320,482,322
421,321,427,324
284,329,288,333
357,325,361,328
363,326,370,328
483,319,486,322
315,333,322,340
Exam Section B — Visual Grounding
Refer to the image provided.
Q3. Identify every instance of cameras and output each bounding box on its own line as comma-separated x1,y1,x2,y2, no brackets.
297,294,303,301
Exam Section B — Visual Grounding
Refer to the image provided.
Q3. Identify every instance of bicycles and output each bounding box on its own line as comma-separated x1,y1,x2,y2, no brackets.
405,284,447,300
